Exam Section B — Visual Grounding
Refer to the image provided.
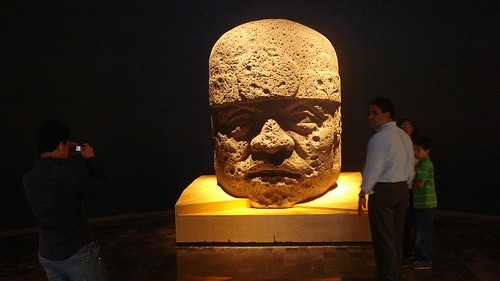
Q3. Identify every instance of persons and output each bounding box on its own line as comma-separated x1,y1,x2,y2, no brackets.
396,116,419,268
208,18,342,207
22,119,110,281
358,96,415,281
412,138,438,270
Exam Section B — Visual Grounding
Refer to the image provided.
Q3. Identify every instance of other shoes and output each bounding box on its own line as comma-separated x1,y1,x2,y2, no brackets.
401,253,415,268
409,259,433,270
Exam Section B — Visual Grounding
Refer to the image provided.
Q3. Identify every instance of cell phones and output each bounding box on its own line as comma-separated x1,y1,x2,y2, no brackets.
75,145,86,152
